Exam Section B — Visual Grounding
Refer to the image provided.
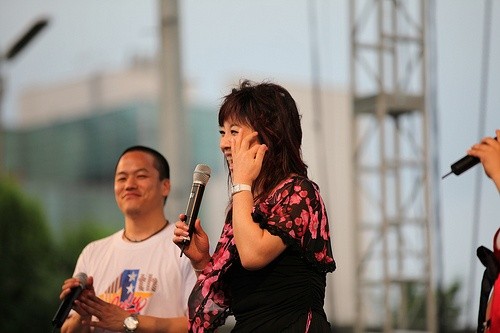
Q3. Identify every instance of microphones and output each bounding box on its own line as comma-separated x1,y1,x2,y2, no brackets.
50,272,88,333
179,164,211,258
442,137,497,180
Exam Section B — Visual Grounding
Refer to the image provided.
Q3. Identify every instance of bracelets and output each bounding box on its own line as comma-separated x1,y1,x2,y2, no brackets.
193,265,206,274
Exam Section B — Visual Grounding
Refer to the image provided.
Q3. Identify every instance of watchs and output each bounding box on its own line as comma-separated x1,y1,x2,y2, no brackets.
122,311,140,333
230,182,252,196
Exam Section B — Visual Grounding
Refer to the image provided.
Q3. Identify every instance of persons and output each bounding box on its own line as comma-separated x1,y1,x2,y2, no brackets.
468,129,500,333
173,79,337,333
60,145,197,333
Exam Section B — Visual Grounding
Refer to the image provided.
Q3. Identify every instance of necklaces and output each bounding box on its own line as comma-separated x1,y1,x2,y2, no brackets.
122,219,169,242
